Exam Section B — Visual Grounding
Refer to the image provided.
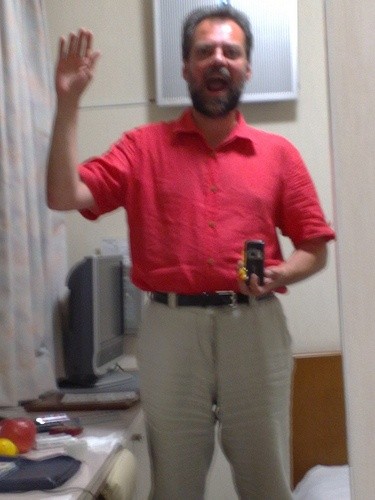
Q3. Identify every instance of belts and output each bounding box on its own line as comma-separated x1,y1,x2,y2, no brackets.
149,290,274,308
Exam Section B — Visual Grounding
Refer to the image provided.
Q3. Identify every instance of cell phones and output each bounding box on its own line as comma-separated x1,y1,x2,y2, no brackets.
244,239,265,286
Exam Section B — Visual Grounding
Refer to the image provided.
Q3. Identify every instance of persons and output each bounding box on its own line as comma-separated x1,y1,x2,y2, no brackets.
44,3,336,500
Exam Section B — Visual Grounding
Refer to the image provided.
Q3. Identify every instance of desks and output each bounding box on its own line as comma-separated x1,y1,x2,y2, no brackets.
0,379,143,499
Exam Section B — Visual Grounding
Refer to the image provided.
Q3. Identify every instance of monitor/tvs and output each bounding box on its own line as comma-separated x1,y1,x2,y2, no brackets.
66,253,135,387
